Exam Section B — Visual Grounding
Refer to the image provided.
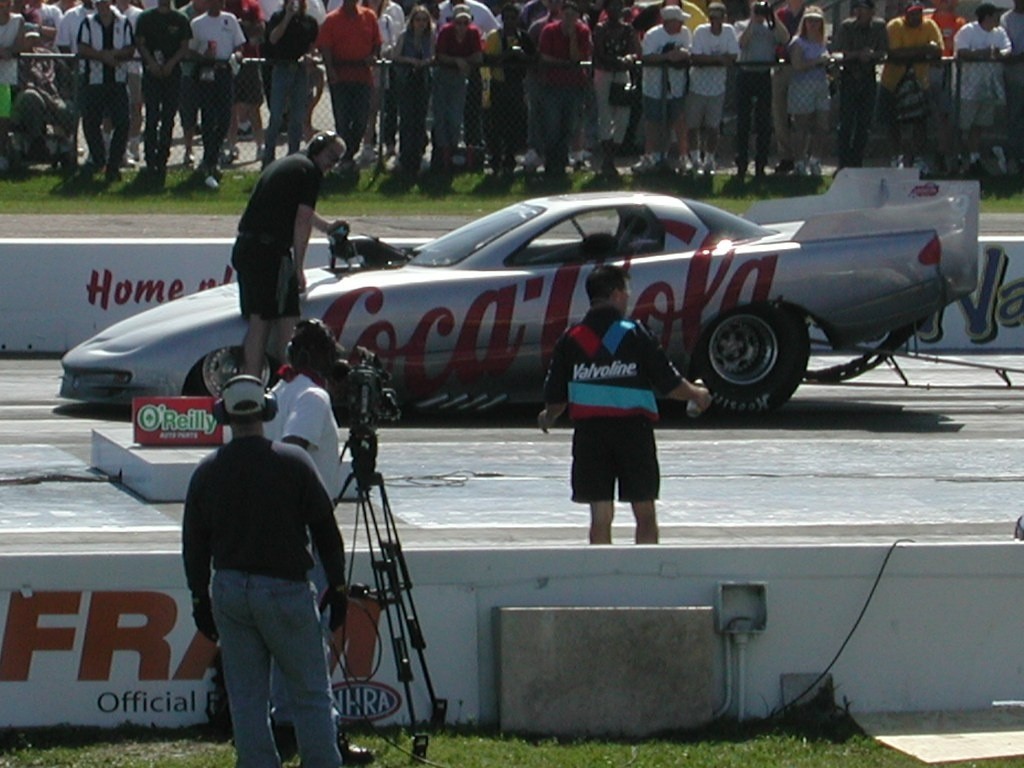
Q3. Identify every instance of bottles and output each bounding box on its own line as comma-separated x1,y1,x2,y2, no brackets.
687,379,705,415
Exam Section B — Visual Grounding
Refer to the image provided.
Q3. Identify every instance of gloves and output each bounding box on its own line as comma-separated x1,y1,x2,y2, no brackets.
192,595,220,642
319,585,348,632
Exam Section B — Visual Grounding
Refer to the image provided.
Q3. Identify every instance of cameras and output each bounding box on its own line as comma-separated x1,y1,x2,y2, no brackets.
755,2,771,17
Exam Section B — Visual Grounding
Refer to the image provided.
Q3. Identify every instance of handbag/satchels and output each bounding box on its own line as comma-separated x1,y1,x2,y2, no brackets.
608,63,637,107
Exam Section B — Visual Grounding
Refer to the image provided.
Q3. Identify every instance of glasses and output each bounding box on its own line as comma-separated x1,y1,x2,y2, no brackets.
325,143,341,164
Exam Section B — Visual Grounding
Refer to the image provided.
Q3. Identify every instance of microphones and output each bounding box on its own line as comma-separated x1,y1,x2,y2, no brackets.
382,387,401,415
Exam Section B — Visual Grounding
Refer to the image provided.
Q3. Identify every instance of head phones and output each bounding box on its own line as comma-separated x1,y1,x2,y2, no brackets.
212,375,277,425
309,132,339,155
285,320,334,370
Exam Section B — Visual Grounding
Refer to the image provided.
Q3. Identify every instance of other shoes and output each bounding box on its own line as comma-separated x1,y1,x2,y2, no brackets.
77,125,1024,190
344,743,373,764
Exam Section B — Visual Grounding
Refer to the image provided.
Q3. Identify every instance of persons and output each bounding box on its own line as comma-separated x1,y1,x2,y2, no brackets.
182,375,346,768
0,0,1024,186
261,318,374,765
538,265,712,546
231,131,347,378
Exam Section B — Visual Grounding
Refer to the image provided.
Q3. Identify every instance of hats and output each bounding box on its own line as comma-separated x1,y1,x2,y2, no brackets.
660,6,692,21
852,1,874,9
221,375,266,416
803,12,823,20
905,2,923,15
976,3,1010,16
23,21,40,38
452,4,471,21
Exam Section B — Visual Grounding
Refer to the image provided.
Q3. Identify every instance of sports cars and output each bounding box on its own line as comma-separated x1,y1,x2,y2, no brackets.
56,166,984,420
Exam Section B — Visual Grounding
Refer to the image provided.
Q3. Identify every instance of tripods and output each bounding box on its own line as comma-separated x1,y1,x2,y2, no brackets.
324,434,448,766
